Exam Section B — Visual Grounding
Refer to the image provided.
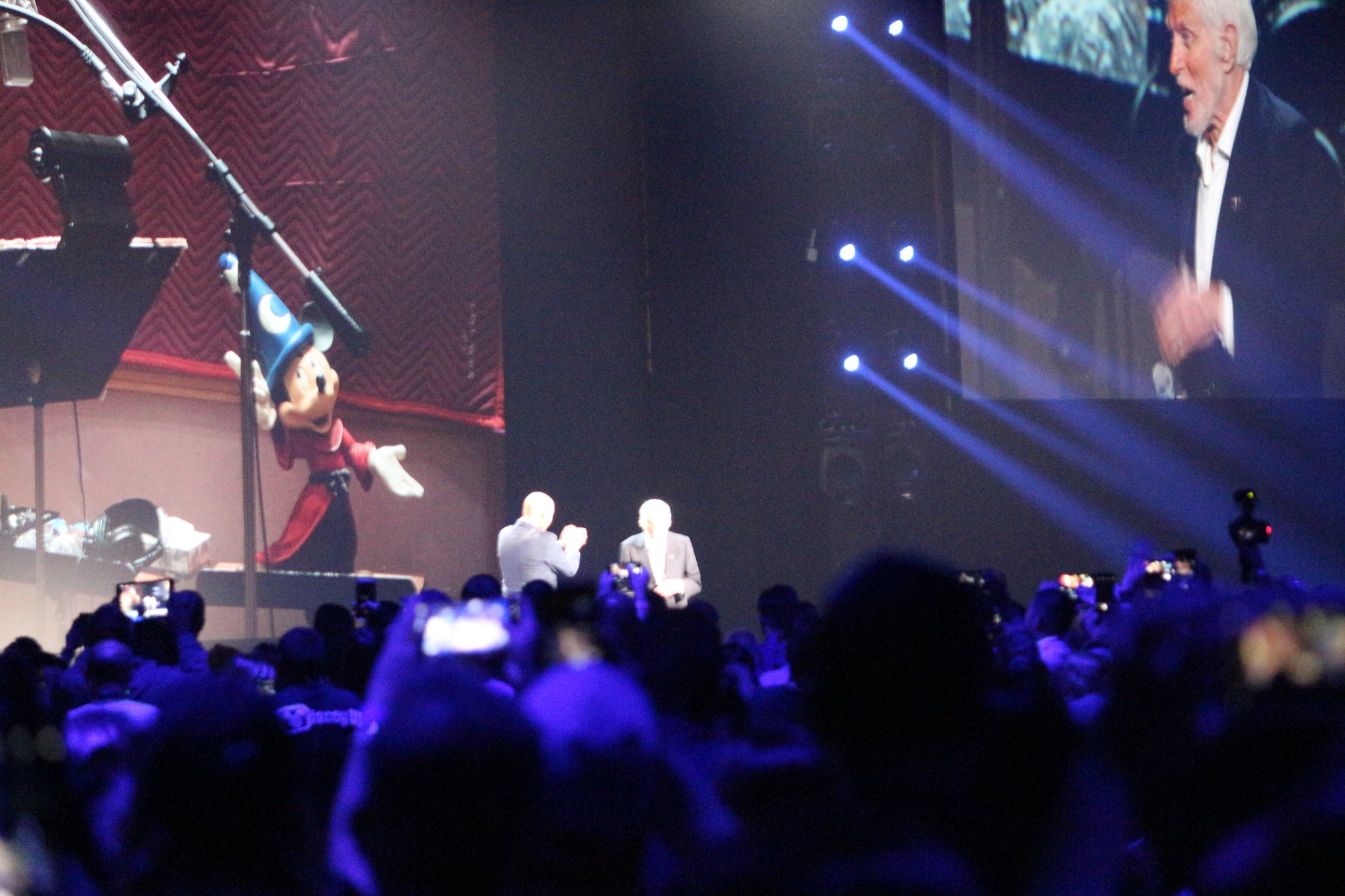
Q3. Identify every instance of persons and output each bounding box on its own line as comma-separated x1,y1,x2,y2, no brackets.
1147,0,1345,402
0,547,1345,896
618,498,702,612
498,490,587,598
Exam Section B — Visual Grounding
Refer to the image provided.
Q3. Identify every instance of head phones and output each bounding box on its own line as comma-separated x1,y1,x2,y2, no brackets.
82,495,165,582
